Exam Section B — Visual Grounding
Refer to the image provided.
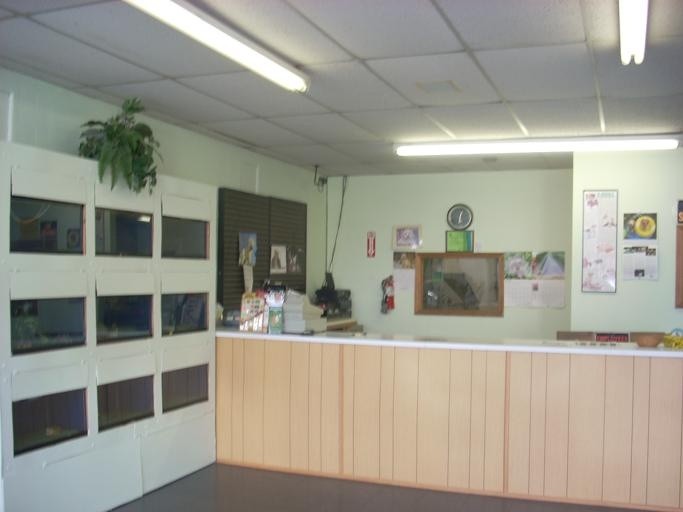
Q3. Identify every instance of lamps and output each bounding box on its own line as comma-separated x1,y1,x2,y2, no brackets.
124,0,310,95
618,1,650,65
393,134,682,158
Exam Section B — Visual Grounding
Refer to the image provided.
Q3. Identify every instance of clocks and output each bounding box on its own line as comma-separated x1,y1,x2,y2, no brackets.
447,203,473,231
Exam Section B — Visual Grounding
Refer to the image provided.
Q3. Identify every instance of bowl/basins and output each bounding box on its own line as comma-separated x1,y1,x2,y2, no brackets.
632,332,666,347
